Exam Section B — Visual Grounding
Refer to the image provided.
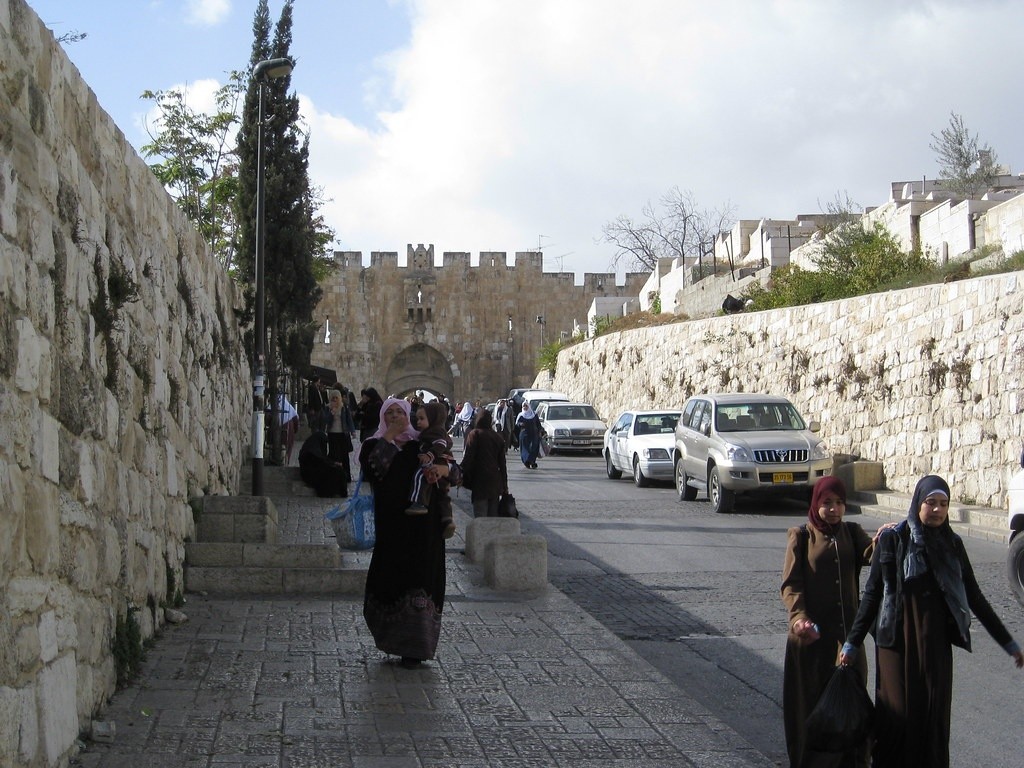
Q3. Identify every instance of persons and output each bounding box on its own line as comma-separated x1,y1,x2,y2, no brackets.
838,476,1024,768
266,375,548,669
779,475,898,768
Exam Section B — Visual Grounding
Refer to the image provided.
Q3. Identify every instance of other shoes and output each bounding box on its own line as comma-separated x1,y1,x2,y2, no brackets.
532,463,537,468
405,503,428,515
402,656,421,669
441,522,455,539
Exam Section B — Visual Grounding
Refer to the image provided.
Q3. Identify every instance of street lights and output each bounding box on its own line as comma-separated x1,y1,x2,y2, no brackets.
252,56,294,499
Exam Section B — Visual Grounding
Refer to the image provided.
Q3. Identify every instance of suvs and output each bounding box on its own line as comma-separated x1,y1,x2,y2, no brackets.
673,393,833,513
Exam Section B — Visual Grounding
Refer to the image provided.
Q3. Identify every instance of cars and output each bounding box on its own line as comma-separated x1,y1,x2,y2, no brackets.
602,409,709,488
484,388,547,415
534,401,608,457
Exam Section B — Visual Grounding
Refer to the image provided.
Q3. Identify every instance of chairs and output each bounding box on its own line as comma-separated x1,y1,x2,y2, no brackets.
716,413,777,429
640,421,649,431
548,410,560,418
572,410,583,418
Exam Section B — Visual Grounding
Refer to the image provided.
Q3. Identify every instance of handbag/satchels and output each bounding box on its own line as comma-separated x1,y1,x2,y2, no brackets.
805,664,874,754
325,495,376,550
539,438,549,457
462,463,473,489
499,491,519,518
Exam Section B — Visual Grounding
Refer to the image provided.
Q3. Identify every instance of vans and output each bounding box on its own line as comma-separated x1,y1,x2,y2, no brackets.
522,391,571,417
